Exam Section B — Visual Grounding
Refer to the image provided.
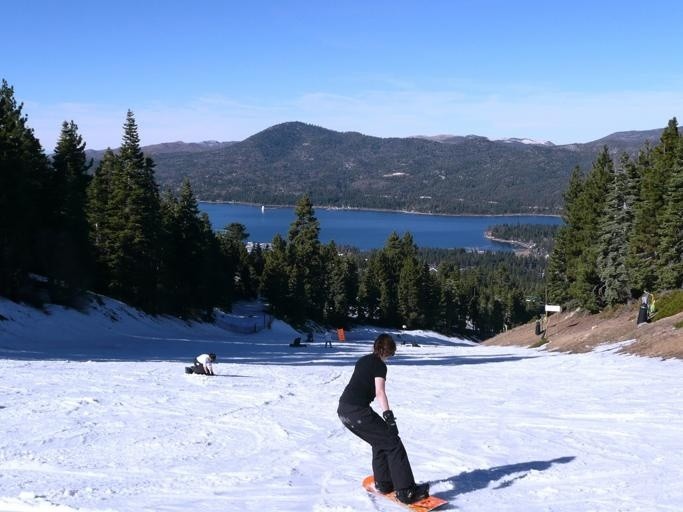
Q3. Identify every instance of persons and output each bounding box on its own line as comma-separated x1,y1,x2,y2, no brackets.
306,331,314,342
324,329,332,348
337,334,429,504
294,336,301,347
189,352,216,375
543,313,548,330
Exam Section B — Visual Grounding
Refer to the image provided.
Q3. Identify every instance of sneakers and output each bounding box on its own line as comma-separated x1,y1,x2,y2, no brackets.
395,482,431,503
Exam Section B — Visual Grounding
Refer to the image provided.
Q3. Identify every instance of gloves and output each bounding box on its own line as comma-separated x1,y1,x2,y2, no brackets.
382,410,400,439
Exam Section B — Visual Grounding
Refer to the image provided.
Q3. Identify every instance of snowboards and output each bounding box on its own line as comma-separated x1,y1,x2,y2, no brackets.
363,476,448,512
